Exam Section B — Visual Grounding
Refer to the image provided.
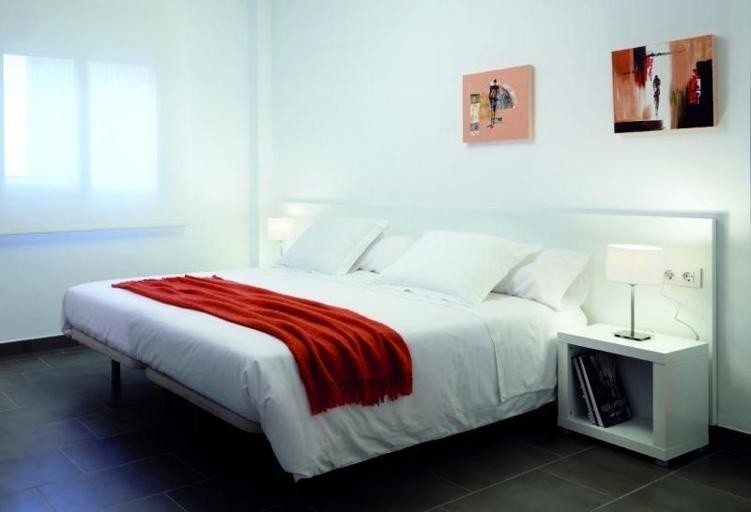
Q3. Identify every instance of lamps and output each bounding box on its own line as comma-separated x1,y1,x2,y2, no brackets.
606,245,665,340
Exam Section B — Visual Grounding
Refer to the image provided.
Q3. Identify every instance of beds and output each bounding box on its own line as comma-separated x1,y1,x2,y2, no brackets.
62,201,719,482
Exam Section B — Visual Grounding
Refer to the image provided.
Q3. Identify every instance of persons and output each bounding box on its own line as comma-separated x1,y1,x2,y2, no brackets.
653,75,660,115
489,80,499,127
687,69,702,105
470,96,480,135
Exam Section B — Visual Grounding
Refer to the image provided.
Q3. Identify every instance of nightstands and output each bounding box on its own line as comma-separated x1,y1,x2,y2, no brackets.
557,324,709,461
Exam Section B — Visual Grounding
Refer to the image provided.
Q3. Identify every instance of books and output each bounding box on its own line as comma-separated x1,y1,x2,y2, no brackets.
571,352,634,428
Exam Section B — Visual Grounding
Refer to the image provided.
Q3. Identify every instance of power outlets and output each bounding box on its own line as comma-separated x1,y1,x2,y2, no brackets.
665,268,702,288
